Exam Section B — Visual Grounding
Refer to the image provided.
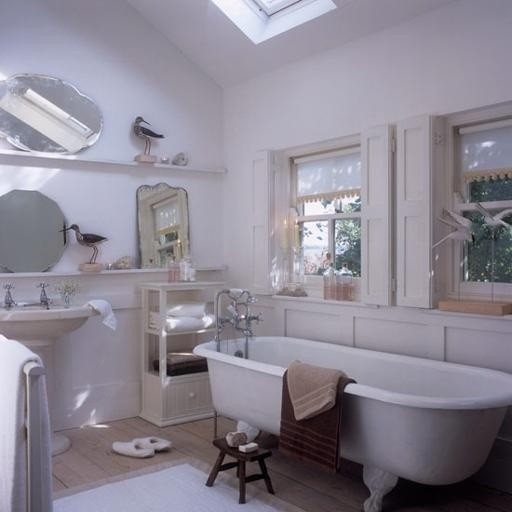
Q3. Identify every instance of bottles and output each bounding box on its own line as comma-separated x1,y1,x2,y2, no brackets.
181,256,198,282
323,262,337,300
337,263,353,300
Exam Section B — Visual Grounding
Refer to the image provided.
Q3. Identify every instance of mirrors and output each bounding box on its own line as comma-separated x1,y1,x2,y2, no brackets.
0,190,71,272
136,182,191,270
0,72,104,156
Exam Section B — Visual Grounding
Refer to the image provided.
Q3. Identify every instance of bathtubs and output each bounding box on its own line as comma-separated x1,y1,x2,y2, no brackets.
193,336,512,486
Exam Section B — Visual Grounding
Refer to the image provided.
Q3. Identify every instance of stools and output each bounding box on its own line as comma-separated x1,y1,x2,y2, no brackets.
204,437,274,506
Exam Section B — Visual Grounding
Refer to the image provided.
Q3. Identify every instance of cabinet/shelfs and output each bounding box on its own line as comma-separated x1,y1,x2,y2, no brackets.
138,280,224,429
0,149,228,280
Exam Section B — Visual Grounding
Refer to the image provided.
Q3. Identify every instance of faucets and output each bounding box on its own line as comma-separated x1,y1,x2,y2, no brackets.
35,281,51,304
3,283,18,308
233,324,254,337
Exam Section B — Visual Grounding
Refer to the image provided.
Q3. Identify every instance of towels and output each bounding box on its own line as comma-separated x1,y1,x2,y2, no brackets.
148,301,219,333
82,299,117,332
277,359,357,475
1,334,54,512
153,349,208,377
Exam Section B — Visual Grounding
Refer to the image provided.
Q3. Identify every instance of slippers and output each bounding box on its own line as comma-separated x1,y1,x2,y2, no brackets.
112,441,155,458
133,437,172,451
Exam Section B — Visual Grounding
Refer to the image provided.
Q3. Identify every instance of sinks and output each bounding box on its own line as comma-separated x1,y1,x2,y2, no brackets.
1,304,92,341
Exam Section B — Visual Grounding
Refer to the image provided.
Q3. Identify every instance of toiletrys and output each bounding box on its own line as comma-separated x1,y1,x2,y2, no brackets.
180,256,188,282
189,266,197,282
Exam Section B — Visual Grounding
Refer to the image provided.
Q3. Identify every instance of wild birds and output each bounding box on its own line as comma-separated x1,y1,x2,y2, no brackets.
60,224,111,265
132,116,167,154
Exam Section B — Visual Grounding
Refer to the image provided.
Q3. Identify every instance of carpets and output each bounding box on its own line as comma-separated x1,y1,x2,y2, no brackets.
52,453,309,512
